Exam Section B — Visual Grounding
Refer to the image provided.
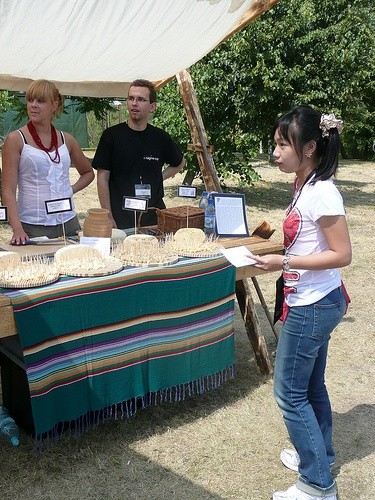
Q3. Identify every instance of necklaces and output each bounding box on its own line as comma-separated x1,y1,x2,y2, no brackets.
27,120,60,163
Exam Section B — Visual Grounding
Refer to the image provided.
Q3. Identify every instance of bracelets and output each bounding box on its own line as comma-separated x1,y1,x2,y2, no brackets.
282,256,291,272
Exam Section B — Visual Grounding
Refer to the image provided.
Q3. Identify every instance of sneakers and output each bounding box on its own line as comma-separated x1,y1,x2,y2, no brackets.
281,448,335,476
272,484,339,500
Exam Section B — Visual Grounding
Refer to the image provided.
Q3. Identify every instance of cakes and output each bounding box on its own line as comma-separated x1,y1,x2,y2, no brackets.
0,251,22,277
173,227,205,248
121,234,159,258
53,244,102,272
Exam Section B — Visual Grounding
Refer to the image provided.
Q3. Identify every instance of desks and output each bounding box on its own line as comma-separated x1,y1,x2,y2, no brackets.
0,225,285,451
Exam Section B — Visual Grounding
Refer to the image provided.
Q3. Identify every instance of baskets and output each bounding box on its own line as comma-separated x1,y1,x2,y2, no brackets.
156,206,206,235
137,207,165,241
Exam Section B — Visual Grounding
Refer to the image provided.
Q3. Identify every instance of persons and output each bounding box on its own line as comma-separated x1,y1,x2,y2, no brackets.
91,78,185,230
1,79,95,246
244,103,352,500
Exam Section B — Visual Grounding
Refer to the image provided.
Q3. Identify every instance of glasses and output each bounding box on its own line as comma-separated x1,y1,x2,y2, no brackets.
126,96,150,104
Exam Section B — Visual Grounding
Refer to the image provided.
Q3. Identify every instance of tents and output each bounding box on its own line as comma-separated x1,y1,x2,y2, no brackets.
0,0,279,377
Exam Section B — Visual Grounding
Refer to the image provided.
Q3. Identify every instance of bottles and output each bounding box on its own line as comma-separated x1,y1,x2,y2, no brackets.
205,199,216,238
199,191,209,208
0,406,19,445
84,208,112,237
